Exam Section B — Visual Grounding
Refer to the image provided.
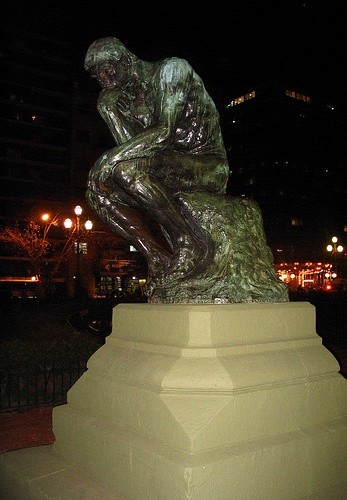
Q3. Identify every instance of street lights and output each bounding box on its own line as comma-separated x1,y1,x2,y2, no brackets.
62,204,93,307
324,236,344,290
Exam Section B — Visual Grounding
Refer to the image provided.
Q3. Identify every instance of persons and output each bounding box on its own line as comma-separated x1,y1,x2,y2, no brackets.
83,36,230,296
